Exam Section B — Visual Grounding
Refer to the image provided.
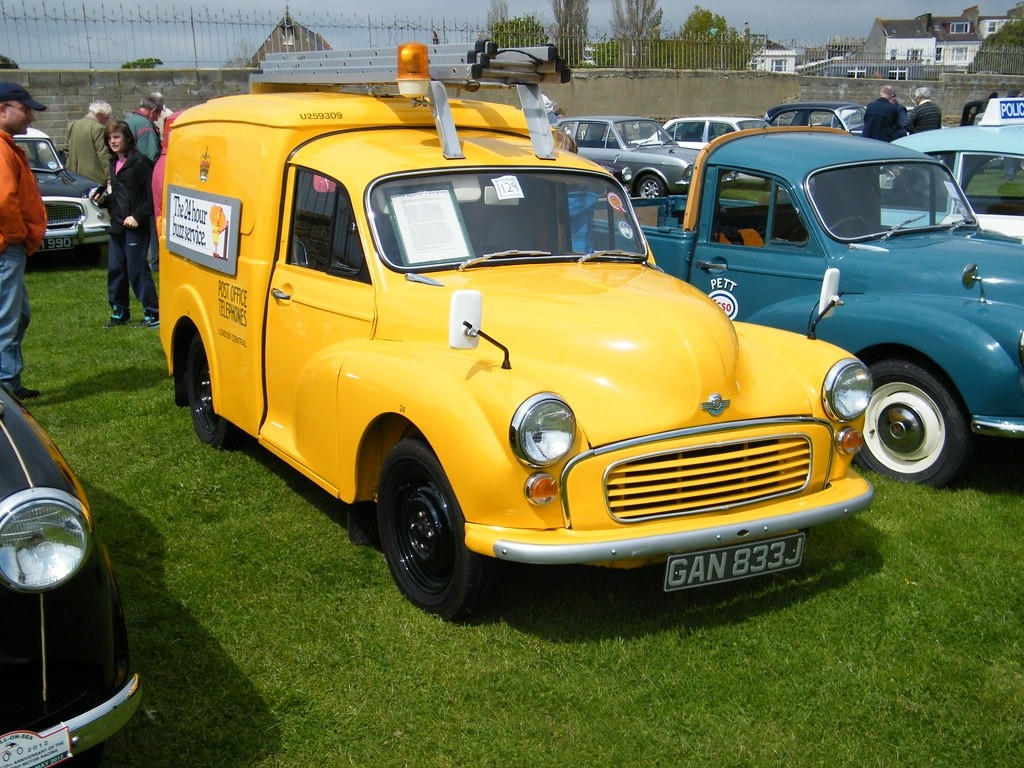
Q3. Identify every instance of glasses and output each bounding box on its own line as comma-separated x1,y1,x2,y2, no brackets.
4,103,32,113
892,93,896,98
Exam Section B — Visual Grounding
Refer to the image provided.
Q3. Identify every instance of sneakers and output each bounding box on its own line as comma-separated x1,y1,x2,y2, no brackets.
103,305,131,329
132,312,160,330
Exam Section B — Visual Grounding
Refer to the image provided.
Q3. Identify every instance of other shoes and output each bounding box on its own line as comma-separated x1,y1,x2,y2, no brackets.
13,386,42,399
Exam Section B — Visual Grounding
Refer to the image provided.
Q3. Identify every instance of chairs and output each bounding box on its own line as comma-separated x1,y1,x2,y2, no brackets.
716,225,764,247
892,170,930,209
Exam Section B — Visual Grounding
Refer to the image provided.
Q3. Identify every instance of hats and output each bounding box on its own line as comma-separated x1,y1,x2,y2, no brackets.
0,82,47,111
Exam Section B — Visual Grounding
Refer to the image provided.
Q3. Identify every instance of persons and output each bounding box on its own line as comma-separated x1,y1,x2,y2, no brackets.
151,111,187,240
1002,89,1023,183
65,100,113,185
125,97,163,163
551,127,599,253
91,121,159,328
151,91,174,147
0,82,48,399
540,92,565,123
959,91,998,126
860,85,940,161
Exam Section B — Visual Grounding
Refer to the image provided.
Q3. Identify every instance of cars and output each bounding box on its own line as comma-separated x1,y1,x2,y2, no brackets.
764,102,868,138
558,113,702,200
0,374,151,768
0,120,113,263
579,128,1024,491
627,118,772,154
871,125,1024,244
154,41,882,625
